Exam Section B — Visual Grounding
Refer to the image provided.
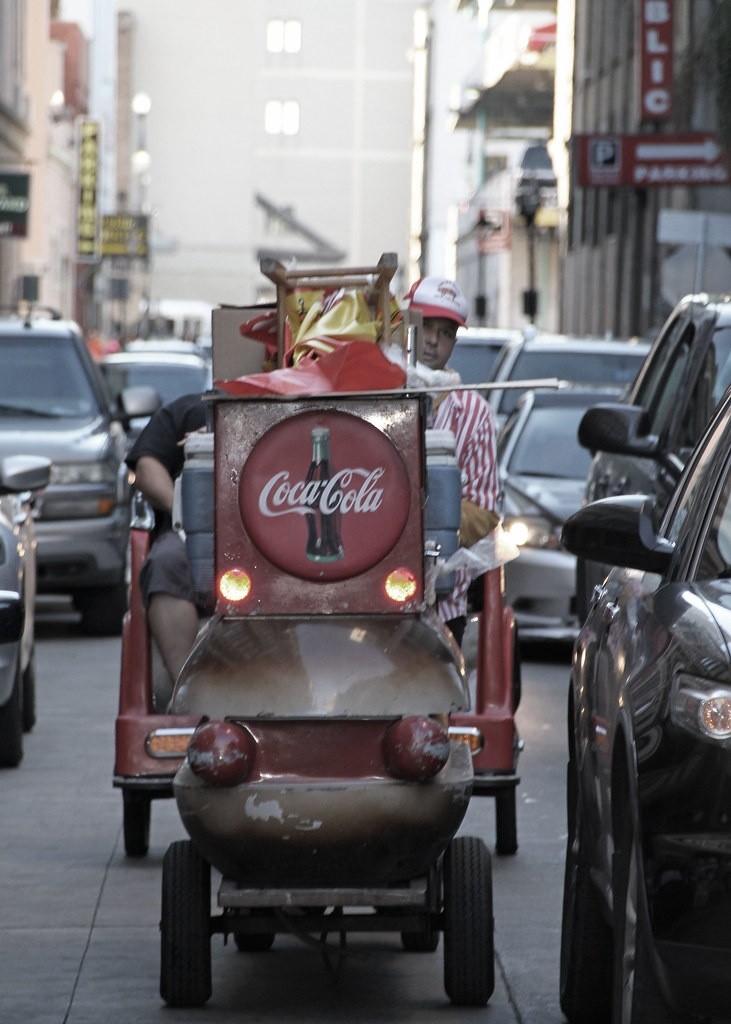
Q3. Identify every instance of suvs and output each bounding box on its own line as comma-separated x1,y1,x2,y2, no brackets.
1,305,213,772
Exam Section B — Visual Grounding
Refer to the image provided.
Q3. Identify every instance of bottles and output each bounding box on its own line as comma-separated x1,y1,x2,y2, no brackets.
424,429,460,593
303,426,344,561
182,431,216,593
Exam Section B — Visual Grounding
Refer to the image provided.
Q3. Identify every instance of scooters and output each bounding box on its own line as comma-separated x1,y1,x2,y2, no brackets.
115,252,557,1015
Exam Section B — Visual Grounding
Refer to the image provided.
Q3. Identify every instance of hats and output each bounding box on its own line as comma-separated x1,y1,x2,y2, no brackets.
407,276,468,328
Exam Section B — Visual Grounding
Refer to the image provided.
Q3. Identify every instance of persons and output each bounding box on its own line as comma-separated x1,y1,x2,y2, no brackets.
123,393,204,683
401,276,500,648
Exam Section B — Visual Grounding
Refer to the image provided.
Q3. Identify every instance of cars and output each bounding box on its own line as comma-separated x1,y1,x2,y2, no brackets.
447,292,731,1024
